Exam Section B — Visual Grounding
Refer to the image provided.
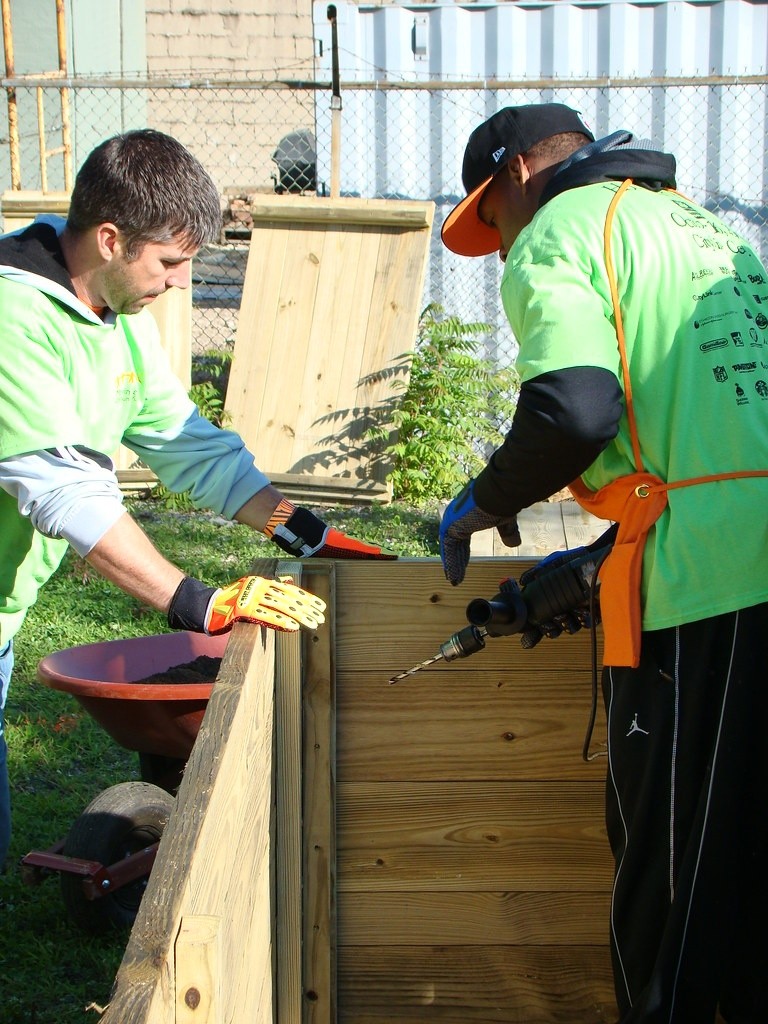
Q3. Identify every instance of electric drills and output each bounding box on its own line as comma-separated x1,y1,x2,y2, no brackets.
387,543,615,689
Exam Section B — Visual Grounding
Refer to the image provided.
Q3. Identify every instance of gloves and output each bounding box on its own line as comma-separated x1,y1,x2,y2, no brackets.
440,477,522,586
168,576,328,637
520,547,601,650
271,507,399,560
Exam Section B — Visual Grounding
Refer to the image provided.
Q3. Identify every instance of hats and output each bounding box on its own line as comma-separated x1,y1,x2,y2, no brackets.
441,103,595,257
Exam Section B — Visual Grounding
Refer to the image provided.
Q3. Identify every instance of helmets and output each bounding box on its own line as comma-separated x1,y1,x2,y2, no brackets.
271,129,316,173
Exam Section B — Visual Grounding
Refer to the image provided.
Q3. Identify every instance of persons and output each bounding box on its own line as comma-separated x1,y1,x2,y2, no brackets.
439,104,767,1024
0,130,398,875
